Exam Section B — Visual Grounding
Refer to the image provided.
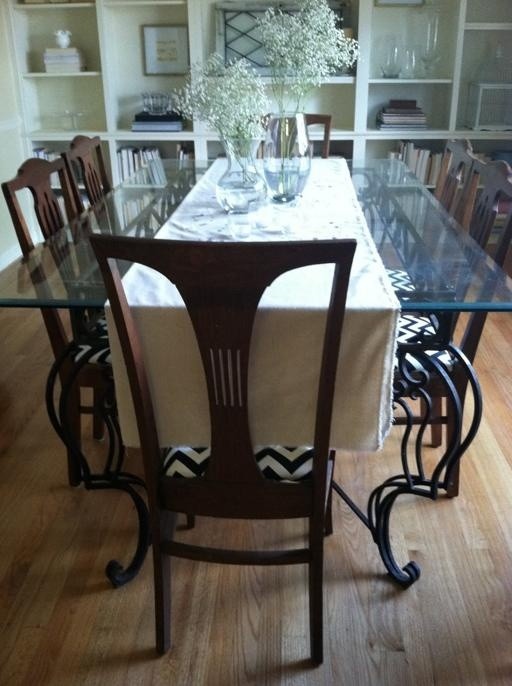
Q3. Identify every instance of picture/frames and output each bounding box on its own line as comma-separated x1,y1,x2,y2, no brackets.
141,25,190,77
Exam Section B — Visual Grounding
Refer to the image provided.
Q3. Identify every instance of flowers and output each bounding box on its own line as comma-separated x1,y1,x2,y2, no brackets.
255,1,360,190
169,53,269,184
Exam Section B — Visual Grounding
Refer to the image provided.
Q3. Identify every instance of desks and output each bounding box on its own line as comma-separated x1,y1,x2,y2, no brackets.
1,158,512,587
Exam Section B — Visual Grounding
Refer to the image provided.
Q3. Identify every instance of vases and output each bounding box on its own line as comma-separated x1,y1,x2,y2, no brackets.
215,138,268,214
263,112,311,202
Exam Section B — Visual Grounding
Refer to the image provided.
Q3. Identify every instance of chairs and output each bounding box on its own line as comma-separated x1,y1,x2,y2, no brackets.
61,135,123,316
254,114,331,159
87,235,359,667
2,157,115,485
385,136,475,418
386,156,512,499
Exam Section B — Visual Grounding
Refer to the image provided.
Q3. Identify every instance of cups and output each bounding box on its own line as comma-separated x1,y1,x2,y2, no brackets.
150,91,172,115
142,91,150,113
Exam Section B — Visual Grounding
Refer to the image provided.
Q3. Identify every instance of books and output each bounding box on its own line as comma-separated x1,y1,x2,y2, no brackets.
375,106,427,131
32,147,61,187
117,144,194,186
123,190,175,230
388,142,443,186
42,47,84,73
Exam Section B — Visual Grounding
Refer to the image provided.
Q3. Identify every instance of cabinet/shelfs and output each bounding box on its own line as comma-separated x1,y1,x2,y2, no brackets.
2,0,512,243
